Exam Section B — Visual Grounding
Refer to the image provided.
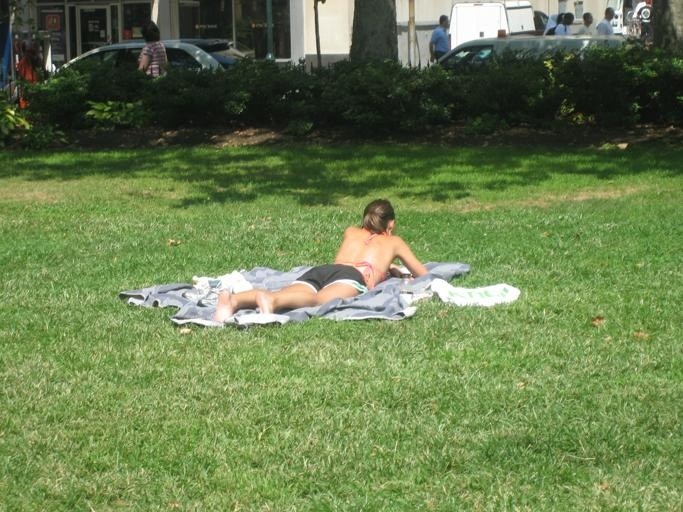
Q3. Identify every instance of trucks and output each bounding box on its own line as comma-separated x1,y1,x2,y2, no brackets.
605,0,652,35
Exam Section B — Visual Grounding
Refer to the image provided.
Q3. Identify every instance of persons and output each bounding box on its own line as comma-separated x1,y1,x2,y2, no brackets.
632,0,654,46
429,15,450,64
542,7,614,36
215,199,429,323
136,20,167,79
15,39,41,109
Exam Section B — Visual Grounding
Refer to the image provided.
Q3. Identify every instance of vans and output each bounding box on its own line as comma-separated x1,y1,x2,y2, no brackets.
429,35,625,68
447,0,548,59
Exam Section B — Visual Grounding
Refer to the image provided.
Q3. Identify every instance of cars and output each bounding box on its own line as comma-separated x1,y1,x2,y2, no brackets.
11,39,255,102
